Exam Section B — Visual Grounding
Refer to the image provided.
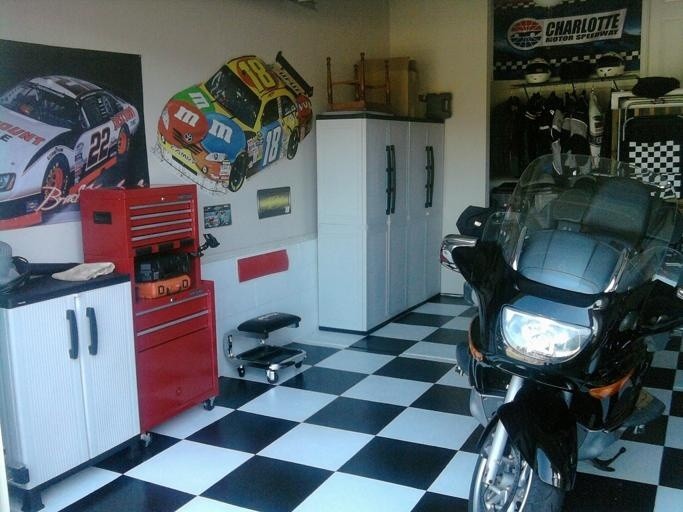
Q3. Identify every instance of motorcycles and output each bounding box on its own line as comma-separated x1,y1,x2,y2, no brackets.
440,152,682,511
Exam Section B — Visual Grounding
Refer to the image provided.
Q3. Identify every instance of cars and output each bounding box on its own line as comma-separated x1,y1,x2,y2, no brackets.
0,72,140,210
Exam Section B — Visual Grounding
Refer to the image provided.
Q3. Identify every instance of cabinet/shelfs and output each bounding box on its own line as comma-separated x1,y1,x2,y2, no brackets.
316,114,444,336
0,274,141,511
79,186,220,449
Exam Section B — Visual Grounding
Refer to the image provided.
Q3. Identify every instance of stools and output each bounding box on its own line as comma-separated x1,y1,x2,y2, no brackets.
324,52,394,111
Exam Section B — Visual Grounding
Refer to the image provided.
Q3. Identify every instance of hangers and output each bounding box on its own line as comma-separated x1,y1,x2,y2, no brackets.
499,85,605,120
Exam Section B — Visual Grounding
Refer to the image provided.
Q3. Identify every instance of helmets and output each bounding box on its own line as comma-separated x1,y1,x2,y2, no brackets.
525,58,551,83
595,51,626,78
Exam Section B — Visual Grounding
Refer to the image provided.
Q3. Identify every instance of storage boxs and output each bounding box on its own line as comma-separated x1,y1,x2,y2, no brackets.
356,57,421,118
